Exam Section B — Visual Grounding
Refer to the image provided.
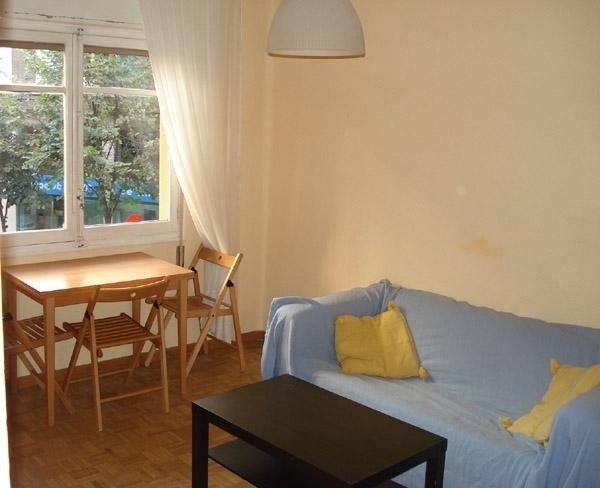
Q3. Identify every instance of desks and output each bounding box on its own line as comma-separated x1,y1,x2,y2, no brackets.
192,373,447,488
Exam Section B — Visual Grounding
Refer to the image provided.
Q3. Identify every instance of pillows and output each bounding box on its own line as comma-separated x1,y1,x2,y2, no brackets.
334,300,430,381
499,358,600,449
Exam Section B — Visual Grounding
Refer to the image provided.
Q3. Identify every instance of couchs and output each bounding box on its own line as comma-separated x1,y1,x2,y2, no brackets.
267,278,599,487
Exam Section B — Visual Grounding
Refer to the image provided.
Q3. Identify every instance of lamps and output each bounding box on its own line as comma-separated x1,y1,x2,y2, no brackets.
267,0,365,59
